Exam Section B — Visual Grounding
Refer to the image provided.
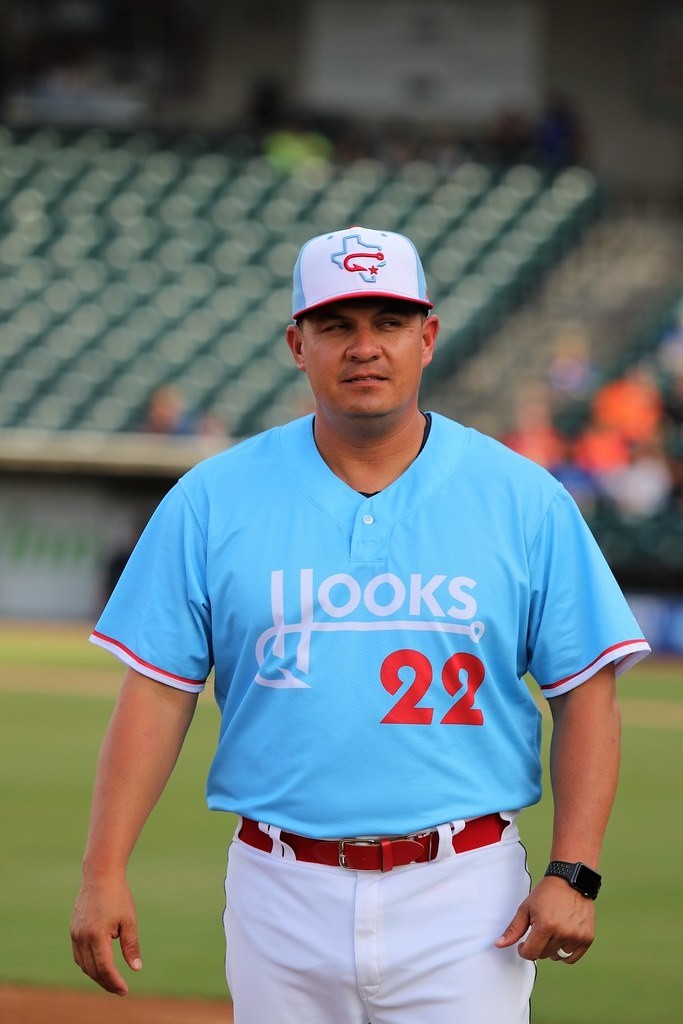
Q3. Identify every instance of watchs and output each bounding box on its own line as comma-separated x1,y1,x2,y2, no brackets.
544,861,603,900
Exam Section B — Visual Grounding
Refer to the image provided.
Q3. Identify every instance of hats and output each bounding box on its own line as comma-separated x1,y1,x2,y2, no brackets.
291,223,433,318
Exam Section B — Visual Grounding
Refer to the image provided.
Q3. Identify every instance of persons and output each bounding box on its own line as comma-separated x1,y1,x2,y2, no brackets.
70,228,652,1024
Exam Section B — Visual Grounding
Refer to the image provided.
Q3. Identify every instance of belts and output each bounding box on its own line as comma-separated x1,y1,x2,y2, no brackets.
239,813,506,872
